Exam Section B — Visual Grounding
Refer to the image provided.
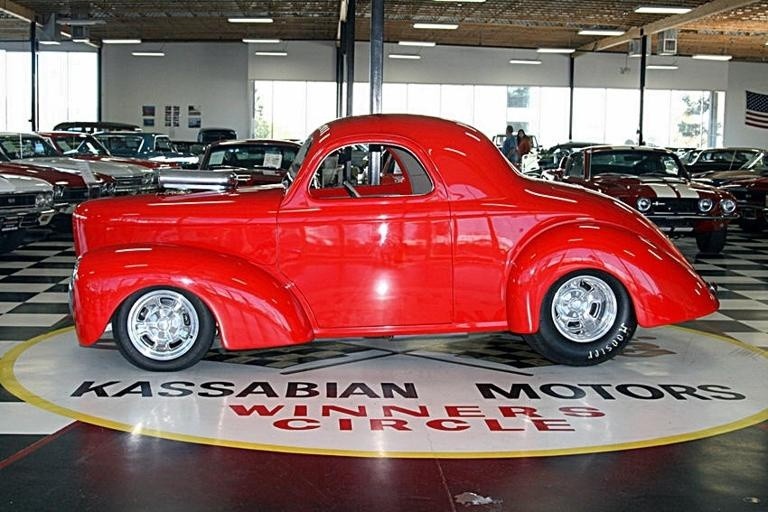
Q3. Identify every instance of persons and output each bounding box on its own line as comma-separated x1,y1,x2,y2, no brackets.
518,129,527,157
502,126,519,167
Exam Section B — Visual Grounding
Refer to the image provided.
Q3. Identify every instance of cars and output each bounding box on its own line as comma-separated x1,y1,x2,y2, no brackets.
68,114,720,372
